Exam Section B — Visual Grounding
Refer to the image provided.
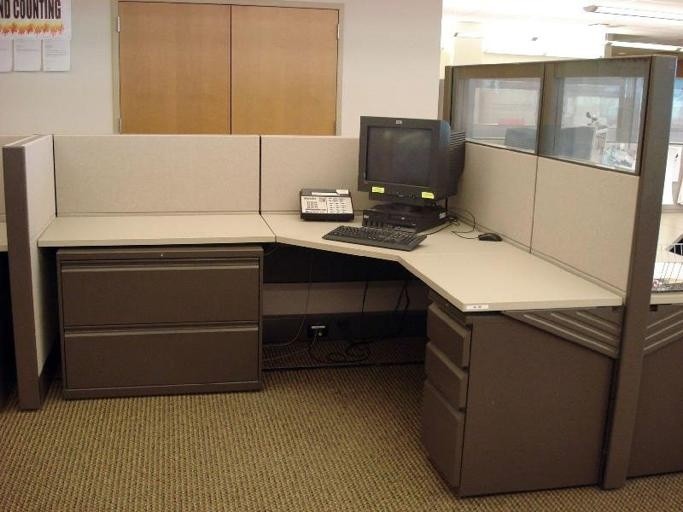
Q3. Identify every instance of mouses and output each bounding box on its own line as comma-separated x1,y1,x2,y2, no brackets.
478,232,502,242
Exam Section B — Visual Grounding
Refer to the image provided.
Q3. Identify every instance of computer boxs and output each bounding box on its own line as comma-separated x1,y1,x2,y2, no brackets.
362,204,447,234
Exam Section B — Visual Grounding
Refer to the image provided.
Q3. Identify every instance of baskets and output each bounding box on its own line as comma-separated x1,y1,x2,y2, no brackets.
651,242,683,292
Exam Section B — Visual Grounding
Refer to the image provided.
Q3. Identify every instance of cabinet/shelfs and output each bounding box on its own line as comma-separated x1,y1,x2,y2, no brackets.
420,288,626,499
56,245,264,401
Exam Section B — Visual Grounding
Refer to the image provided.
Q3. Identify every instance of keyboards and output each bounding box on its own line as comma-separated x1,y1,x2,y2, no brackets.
322,225,427,251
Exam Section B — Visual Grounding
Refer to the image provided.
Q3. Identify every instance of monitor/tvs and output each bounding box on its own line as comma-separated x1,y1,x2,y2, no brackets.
504,126,608,164
357,115,466,212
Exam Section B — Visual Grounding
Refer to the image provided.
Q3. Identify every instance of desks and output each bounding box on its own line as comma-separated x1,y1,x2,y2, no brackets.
35,134,277,248
260,134,640,314
